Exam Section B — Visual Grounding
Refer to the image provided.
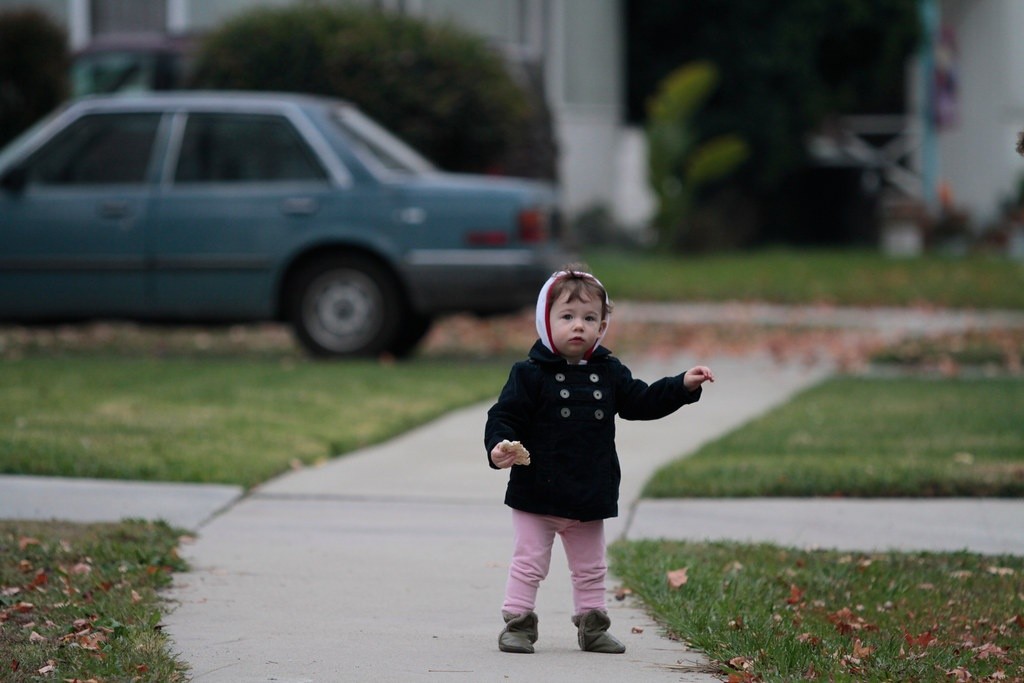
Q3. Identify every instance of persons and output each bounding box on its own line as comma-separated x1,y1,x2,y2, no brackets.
484,270,715,655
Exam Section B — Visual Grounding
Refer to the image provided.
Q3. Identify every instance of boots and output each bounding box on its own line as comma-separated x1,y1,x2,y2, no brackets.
497,612,539,653
571,609,626,653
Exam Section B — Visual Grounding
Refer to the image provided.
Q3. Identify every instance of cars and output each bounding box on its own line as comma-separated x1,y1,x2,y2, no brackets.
0,91,560,363
61,31,214,99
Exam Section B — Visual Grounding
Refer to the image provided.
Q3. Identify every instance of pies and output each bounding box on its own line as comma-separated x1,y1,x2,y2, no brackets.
499,438,530,466
